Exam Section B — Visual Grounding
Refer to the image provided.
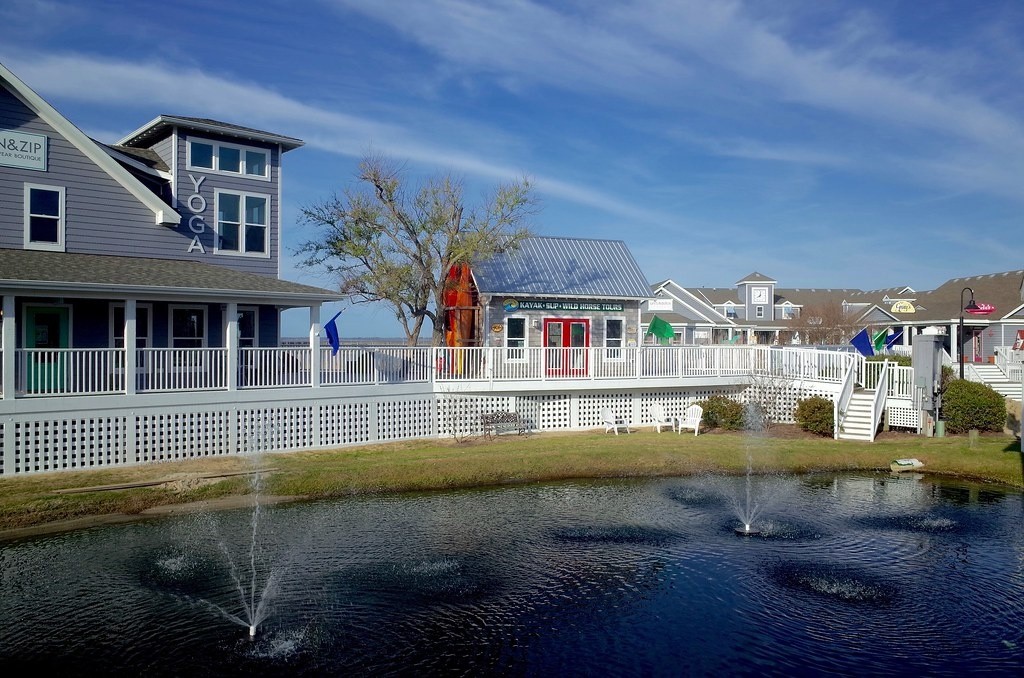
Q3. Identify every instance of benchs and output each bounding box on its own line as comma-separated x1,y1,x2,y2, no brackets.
483,411,528,442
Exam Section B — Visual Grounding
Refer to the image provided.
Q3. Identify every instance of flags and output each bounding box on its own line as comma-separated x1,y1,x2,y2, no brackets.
323,320,340,356
648,316,675,338
850,329,875,357
886,332,901,350
874,329,888,351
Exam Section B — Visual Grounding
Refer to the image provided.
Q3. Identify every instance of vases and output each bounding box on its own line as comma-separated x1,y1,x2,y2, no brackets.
988,357,994,364
964,356,968,362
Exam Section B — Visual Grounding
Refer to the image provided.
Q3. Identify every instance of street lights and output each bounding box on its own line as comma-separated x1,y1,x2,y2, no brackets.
959,287,981,381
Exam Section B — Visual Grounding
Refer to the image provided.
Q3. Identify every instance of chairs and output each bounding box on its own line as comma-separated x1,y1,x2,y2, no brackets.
650,405,676,433
679,404,703,436
601,408,630,435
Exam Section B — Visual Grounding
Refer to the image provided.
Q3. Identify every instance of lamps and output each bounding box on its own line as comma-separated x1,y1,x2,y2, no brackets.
533,320,539,328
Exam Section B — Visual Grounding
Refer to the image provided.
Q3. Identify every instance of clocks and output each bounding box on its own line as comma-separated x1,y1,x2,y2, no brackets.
751,286,768,304
